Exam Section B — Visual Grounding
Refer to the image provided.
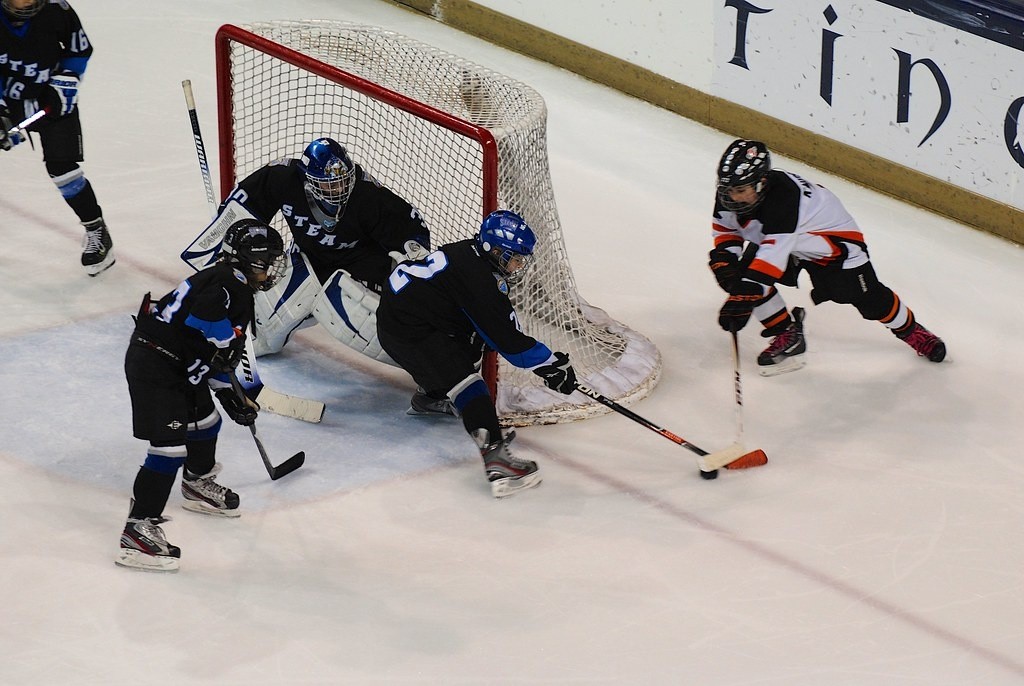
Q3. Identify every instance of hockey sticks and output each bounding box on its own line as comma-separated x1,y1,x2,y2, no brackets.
180,76,329,426
572,381,769,470
699,330,747,474
228,369,306,481
7,107,49,138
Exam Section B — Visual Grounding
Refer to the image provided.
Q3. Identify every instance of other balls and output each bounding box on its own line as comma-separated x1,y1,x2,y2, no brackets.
700,470,719,479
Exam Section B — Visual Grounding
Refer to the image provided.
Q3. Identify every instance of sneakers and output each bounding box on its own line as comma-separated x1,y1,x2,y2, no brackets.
482,429,542,497
759,307,808,376
80,206,116,276
902,322,954,364
406,386,461,418
115,498,182,571
181,462,242,517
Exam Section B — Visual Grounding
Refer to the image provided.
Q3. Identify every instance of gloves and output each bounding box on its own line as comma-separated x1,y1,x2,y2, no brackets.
214,388,260,426
709,254,740,293
533,352,582,395
217,327,248,372
37,74,80,120
0,104,26,151
718,280,764,332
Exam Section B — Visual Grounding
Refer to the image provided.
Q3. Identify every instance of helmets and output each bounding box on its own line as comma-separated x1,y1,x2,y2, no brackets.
222,218,290,291
716,138,771,212
298,139,357,206
476,210,537,280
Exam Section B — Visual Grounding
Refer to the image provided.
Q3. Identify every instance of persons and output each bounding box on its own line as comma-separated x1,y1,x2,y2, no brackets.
708,139,952,377
114,219,286,570
376,210,576,498
181,137,431,369
0,1,116,276
